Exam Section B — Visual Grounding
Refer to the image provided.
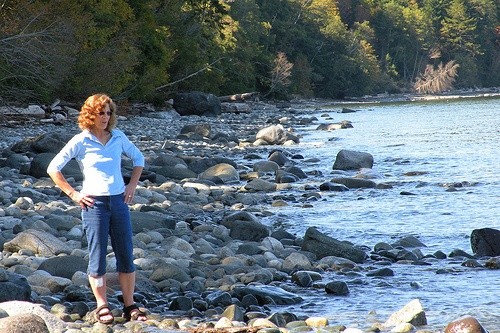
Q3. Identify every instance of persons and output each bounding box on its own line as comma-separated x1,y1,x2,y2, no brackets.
47,93,147,324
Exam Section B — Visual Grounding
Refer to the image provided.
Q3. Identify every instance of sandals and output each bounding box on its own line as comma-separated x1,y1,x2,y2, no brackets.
93,304,115,324
121,304,148,322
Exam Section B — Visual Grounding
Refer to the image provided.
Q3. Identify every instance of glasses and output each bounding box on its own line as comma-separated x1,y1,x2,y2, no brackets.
97,111,112,116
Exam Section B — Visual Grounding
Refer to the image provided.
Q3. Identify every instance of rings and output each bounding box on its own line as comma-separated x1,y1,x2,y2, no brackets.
128,194,132,197
